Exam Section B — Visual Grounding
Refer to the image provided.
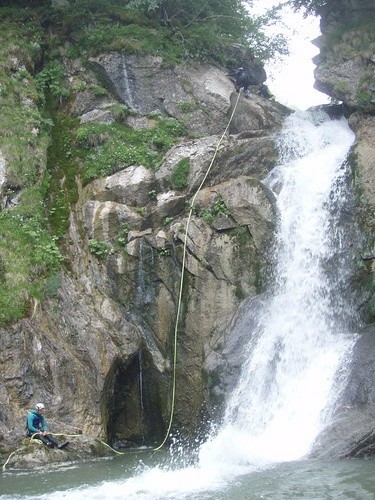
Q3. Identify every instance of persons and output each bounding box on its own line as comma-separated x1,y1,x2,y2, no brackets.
26,403,70,449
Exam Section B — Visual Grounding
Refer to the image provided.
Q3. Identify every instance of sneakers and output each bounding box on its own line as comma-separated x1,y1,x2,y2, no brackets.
58,441,69,449
47,442,55,449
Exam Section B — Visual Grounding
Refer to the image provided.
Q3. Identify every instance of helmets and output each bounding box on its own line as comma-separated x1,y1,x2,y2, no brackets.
35,403,46,410
